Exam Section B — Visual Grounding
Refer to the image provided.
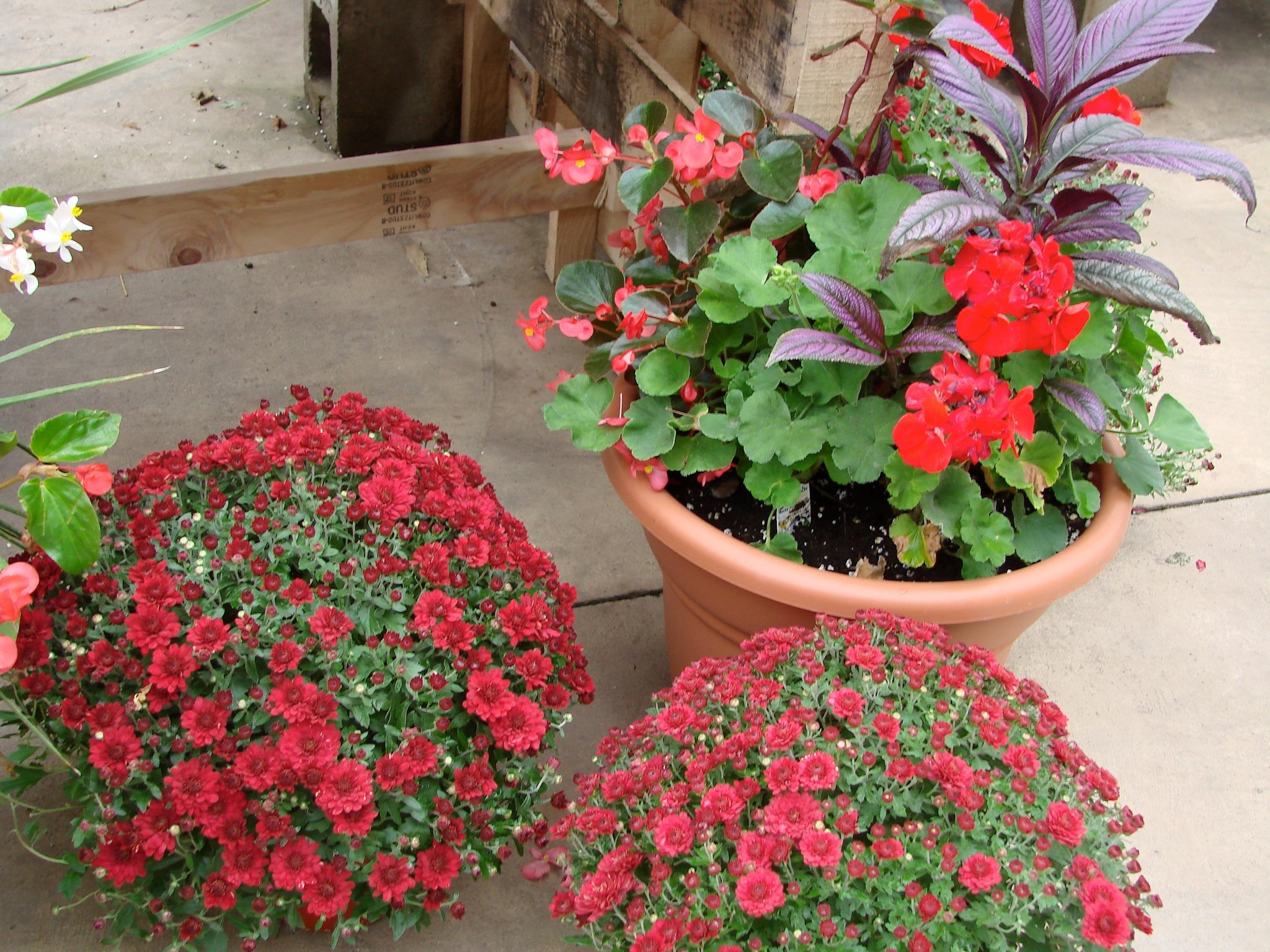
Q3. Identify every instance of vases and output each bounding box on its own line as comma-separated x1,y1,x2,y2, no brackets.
600,374,1133,686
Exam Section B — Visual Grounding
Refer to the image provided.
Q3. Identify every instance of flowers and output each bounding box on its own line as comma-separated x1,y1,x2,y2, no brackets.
523,608,1164,952
518,0,1270,579
0,0,598,952
